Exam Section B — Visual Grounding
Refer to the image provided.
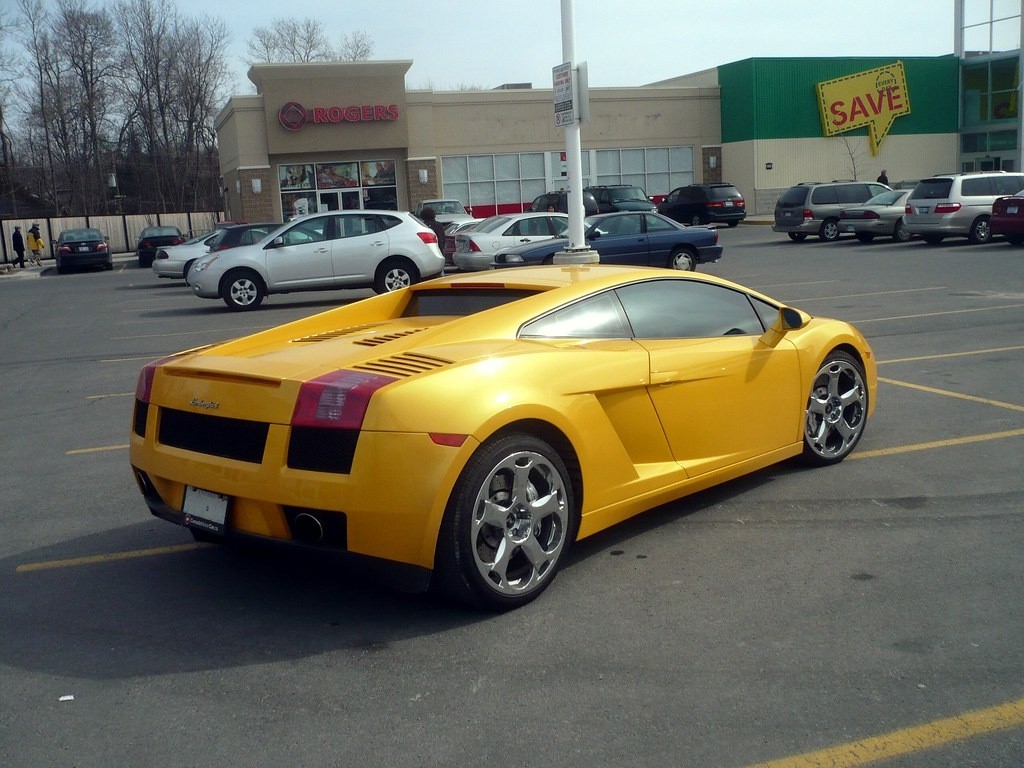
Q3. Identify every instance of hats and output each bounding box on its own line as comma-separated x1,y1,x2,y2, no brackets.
33,223,40,226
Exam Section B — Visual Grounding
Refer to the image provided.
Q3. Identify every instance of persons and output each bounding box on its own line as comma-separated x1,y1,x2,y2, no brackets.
27,223,44,266
320,166,334,187
12,226,25,268
281,166,308,188
547,205,554,212
420,206,445,277
877,169,889,186
374,161,394,185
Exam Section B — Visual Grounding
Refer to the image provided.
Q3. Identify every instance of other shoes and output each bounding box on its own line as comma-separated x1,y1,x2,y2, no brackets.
12,262,16,269
28,259,33,266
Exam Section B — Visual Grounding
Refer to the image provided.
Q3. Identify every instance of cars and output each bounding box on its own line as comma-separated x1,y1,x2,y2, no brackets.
151,227,270,286
660,182,747,228
50,228,113,275
525,189,600,215
134,226,189,268
991,189,1024,245
443,217,489,267
495,211,724,273
208,221,324,254
838,188,917,243
452,212,611,273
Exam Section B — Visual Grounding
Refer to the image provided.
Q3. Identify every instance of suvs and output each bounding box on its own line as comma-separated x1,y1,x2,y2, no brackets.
902,170,1024,246
772,180,896,244
412,199,474,227
186,209,446,312
583,184,658,216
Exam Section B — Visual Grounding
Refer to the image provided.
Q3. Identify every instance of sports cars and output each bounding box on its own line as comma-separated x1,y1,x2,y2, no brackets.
130,263,878,615
